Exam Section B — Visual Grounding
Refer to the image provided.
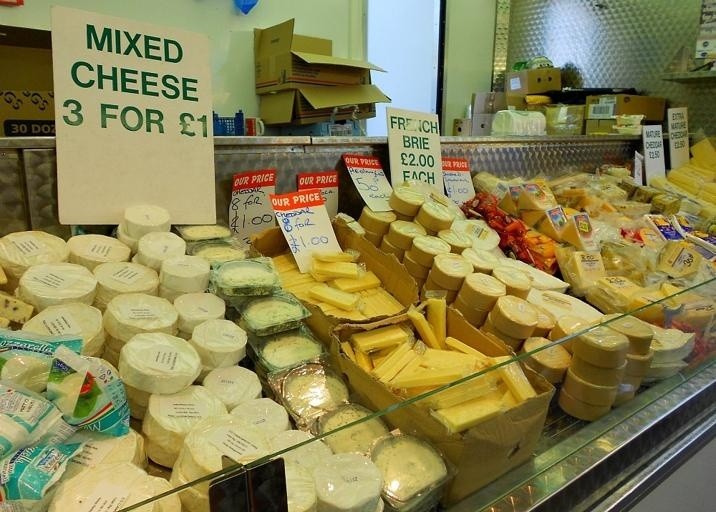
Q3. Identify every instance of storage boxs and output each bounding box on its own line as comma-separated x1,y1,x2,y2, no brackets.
257,82,392,128
324,294,560,508
249,21,388,95
248,215,424,351
0,23,56,137
452,67,668,140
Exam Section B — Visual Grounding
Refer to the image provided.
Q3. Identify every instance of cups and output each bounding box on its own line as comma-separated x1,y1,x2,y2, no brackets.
243,116,264,137
